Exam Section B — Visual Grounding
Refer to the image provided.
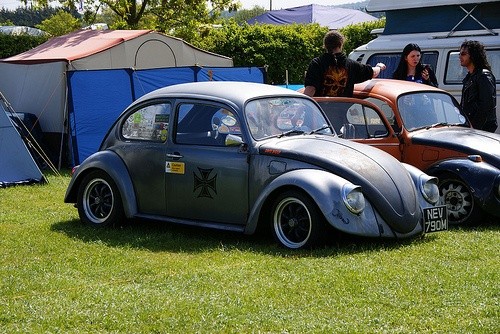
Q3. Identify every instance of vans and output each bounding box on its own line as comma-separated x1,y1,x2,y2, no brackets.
348,0,500,123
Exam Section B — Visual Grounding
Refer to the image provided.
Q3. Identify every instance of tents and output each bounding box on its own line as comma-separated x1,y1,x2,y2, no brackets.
0,27,234,168
240,3,380,30
0,89,65,189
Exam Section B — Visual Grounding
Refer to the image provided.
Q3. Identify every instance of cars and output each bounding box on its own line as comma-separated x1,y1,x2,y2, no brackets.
270,78,500,226
63,81,447,250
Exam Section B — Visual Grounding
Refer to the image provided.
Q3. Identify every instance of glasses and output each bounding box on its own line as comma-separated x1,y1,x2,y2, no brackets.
458,52,469,56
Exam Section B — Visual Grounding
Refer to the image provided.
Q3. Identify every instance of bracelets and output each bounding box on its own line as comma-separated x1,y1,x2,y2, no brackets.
375,65,382,71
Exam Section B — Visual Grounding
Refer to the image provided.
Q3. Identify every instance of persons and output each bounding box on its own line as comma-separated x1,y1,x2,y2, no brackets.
391,43,440,129
291,30,386,138
459,41,498,133
